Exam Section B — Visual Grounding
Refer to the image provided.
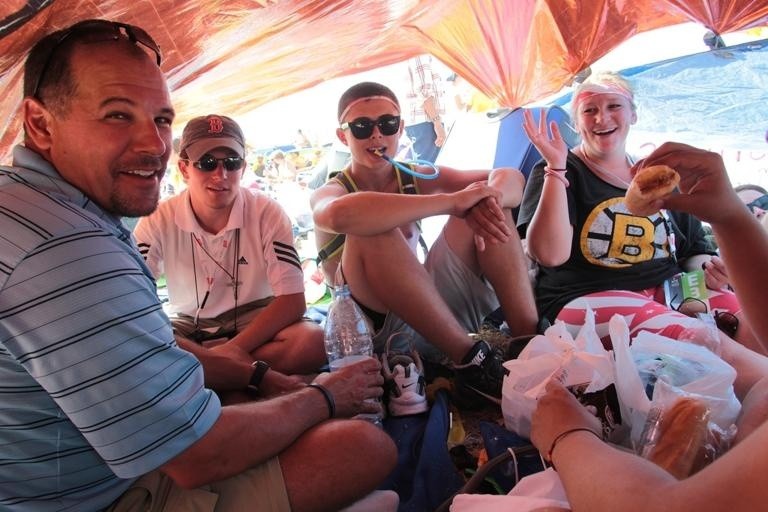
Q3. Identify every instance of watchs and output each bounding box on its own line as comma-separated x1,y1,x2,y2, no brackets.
248,361,272,392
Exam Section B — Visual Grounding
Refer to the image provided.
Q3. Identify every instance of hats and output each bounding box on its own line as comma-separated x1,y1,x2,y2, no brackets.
338,82,400,123
179,115,245,165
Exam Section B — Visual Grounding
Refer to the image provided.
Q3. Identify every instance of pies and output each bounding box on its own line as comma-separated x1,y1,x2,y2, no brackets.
625,164,681,217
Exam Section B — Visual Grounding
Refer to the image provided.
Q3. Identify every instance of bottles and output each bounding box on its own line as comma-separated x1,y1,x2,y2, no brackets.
322,286,386,429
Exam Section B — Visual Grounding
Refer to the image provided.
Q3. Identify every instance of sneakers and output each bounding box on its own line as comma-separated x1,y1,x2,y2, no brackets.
444,341,505,406
380,351,428,416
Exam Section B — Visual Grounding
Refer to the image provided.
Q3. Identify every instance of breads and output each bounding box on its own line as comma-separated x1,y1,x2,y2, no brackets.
649,397,710,474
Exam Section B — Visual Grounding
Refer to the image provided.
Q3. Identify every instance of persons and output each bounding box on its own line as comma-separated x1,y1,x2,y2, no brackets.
255,128,323,176
529,141,768,512
158,138,181,197
311,82,550,402
129,114,324,390
517,73,768,400
3,18,399,512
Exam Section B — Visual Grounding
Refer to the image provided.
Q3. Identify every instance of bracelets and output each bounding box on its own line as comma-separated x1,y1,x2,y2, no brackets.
548,428,601,472
544,166,569,187
306,383,336,418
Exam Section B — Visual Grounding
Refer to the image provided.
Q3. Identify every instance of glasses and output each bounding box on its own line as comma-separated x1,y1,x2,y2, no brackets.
671,292,737,335
746,196,768,212
195,153,242,172
33,18,162,97
339,115,403,140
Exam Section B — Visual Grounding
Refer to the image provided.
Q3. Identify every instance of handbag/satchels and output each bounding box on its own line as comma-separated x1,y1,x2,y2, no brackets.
396,392,525,512
